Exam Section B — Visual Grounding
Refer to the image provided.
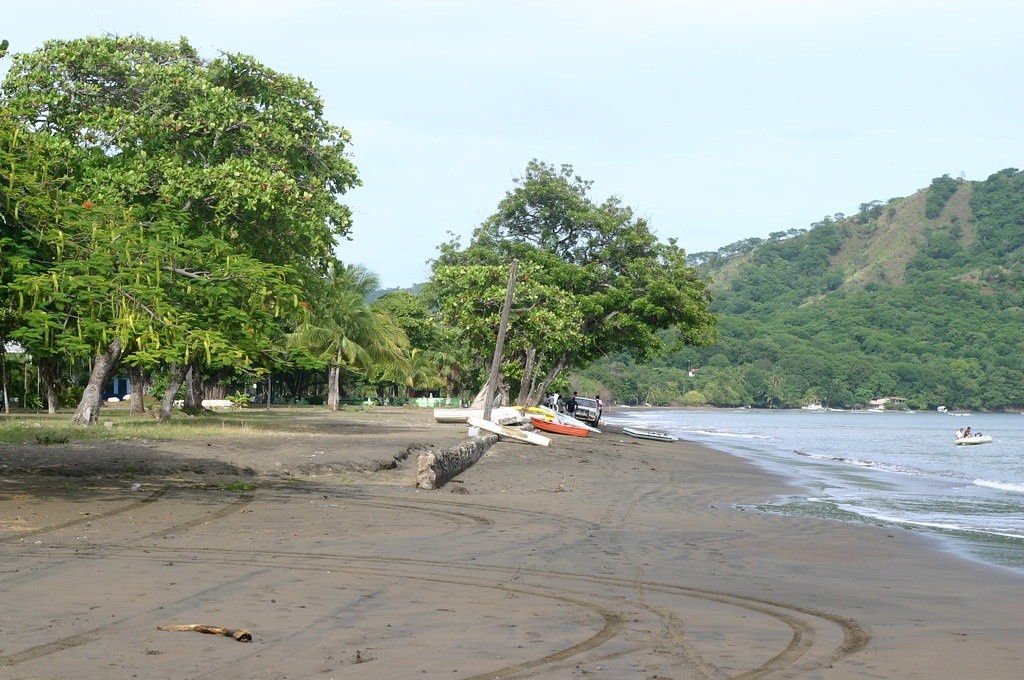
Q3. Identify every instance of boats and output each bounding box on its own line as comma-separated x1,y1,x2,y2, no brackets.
432,404,602,448
622,425,678,442
955,436,992,445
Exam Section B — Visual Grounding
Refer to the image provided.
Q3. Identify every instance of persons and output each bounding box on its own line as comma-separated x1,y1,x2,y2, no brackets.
572,391,578,397
595,395,603,419
545,390,566,414
964,427,971,437
565,397,578,417
956,428,963,439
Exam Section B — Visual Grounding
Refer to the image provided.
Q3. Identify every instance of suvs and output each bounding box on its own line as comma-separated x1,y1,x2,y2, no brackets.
573,396,601,427
